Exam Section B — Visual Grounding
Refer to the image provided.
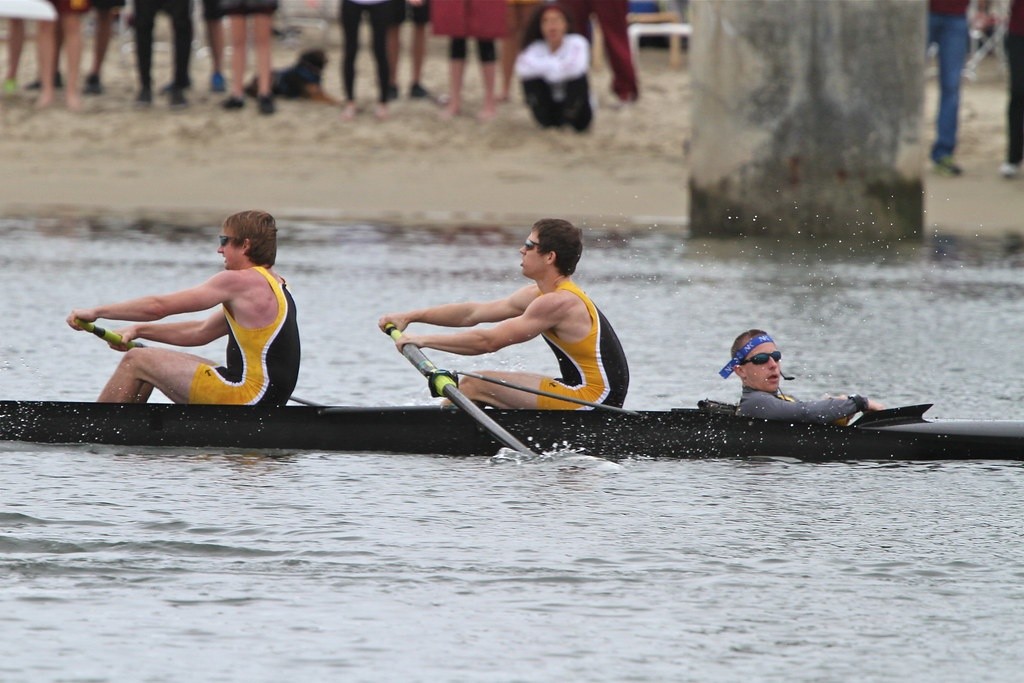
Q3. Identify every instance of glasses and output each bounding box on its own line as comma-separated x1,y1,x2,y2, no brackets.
218,235,234,246
523,238,542,251
741,351,781,365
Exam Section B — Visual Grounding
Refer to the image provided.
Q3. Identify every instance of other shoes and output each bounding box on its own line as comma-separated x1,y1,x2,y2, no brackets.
928,157,961,176
3,64,647,136
1000,161,1017,178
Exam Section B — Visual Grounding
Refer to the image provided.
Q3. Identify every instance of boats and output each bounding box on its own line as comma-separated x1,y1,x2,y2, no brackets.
0,400,1023,461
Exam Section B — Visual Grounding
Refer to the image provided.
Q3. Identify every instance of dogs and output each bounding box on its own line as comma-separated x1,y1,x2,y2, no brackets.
243,47,336,105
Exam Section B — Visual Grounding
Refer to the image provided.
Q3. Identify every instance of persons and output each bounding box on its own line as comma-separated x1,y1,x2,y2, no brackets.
379,219,629,412
928,0,1024,177
66,210,301,406
0,0,641,136
731,330,888,427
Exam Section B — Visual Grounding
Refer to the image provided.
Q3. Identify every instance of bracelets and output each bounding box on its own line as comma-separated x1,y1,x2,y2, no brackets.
848,394,864,412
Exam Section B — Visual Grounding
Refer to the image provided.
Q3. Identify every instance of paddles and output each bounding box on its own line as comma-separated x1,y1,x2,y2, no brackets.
383,321,540,459
73,317,327,407
